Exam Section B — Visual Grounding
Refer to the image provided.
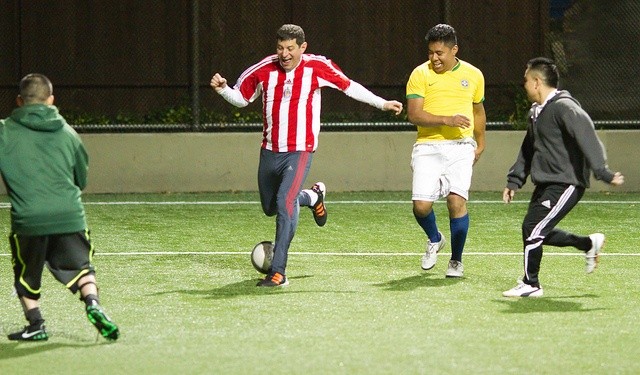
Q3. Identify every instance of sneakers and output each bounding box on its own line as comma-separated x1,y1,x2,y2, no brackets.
420,231,447,269
307,182,327,226
502,279,543,298
256,270,290,288
86,304,121,343
7,323,49,342
445,260,465,279
585,233,604,274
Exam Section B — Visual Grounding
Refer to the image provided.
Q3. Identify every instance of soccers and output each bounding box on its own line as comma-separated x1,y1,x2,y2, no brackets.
251,240,275,275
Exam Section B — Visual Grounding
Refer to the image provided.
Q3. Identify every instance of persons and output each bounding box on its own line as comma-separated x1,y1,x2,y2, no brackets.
209,23,404,291
500,56,627,299
1,72,121,344
402,23,488,280
